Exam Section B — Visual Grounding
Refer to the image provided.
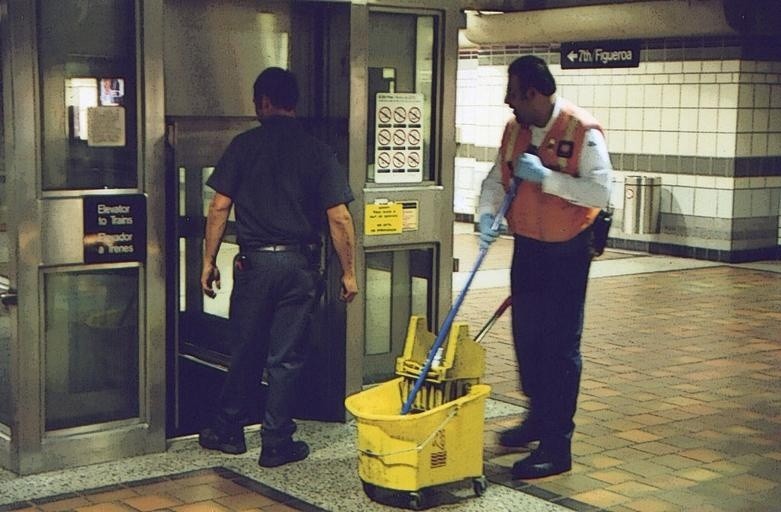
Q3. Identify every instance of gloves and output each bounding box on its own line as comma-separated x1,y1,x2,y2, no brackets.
513,151,550,185
478,214,502,249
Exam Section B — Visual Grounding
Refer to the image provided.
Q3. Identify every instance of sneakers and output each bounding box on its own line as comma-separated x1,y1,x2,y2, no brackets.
500,415,540,445
512,444,572,480
260,439,311,468
200,427,246,453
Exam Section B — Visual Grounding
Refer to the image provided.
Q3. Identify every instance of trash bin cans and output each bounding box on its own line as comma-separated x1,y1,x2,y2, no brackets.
621,175,662,235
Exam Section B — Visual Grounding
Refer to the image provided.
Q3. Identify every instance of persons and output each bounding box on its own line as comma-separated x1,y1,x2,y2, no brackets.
477,56,614,478
199,67,359,466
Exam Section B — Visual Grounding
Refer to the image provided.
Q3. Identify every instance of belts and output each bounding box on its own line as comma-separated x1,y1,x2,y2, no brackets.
254,244,318,255
